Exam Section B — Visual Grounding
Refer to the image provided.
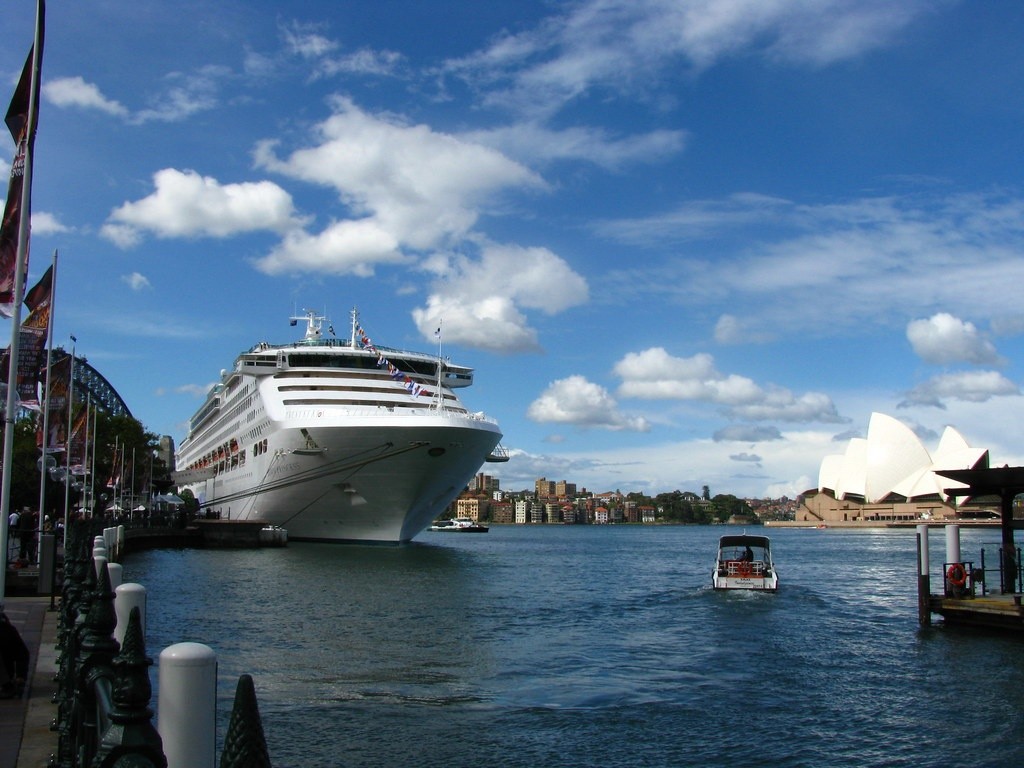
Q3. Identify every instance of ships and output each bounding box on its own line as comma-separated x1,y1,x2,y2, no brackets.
170,309,511,552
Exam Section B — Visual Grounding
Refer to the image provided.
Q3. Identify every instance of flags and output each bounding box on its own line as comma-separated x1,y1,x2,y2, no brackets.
1,4,96,471
354,323,427,400
107,450,152,492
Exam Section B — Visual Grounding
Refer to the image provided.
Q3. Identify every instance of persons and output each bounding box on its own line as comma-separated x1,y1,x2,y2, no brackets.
9,508,79,566
736,547,754,562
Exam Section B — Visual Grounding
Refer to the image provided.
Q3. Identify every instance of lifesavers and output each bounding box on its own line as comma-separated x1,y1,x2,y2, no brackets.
738,561,753,577
947,563,966,587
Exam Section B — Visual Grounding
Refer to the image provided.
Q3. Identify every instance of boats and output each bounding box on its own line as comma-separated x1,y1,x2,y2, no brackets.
189,442,239,472
710,535,781,593
428,517,491,533
